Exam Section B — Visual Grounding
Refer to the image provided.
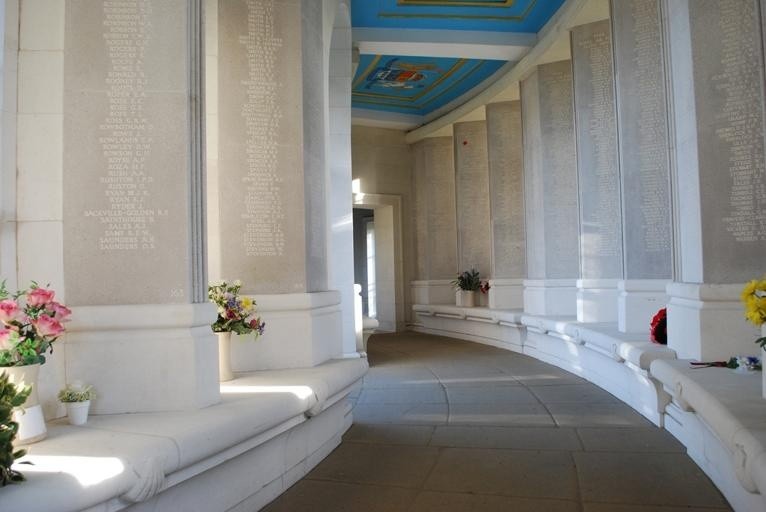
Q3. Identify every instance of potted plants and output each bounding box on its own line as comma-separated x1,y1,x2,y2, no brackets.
450,266,481,308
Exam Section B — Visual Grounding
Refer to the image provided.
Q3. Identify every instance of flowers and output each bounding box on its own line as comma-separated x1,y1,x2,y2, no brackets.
58,380,93,403
645,309,667,342
741,280,765,349
478,279,491,294
208,278,267,344
689,357,760,375
0,278,74,368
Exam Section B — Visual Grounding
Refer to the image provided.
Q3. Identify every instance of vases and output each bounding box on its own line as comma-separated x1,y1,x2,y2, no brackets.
2,363,51,450
65,399,90,426
213,328,237,383
480,292,488,305
